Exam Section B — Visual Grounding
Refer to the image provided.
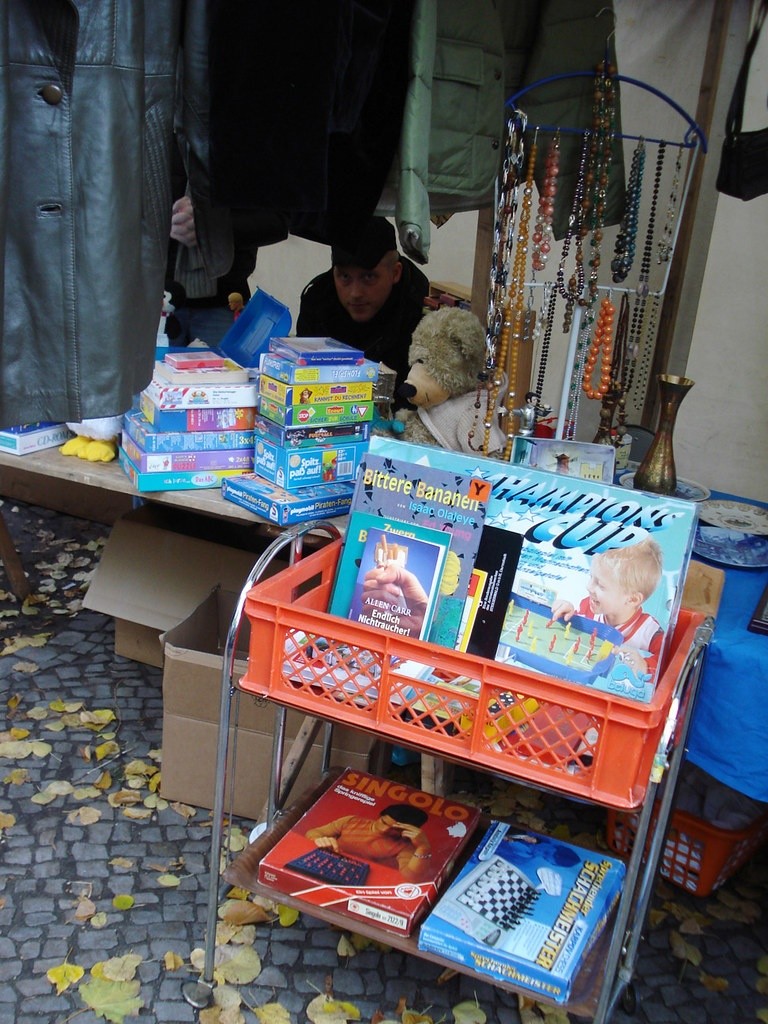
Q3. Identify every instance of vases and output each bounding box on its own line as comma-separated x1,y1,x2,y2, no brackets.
631,373,696,495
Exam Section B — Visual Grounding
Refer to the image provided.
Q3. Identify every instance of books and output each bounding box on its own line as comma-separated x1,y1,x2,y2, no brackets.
274,451,494,718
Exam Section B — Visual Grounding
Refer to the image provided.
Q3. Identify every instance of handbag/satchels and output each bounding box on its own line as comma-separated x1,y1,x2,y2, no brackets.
716,130,768,200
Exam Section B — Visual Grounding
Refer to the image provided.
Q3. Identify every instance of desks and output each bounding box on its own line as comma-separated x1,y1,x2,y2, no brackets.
0,450,352,601
616,463,768,806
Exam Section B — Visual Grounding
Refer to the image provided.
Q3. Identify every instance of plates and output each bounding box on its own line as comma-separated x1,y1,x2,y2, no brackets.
700,500,768,535
692,526,768,567
619,472,711,502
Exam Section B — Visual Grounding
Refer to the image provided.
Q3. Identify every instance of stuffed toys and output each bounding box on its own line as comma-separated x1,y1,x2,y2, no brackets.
393,304,506,462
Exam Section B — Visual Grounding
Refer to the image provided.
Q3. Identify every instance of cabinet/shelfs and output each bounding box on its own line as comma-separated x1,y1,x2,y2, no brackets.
183,525,717,1024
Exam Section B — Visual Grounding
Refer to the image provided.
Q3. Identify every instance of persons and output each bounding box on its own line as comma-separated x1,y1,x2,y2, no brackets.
497,393,548,439
550,533,663,681
227,292,246,321
503,826,540,845
484,824,581,869
360,562,428,640
166,177,260,353
305,803,433,876
296,215,431,418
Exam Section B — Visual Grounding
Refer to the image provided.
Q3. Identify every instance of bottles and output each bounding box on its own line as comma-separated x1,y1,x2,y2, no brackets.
632,373,695,496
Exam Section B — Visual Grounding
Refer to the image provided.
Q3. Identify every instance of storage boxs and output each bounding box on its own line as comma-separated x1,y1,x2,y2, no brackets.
143,369,258,411
119,448,254,494
253,436,370,489
262,352,380,385
270,336,366,366
79,501,321,670
256,767,482,940
222,473,357,527
0,427,76,456
124,410,254,452
161,583,378,828
256,374,374,406
254,415,369,449
608,799,768,898
418,819,627,1002
139,392,256,432
256,395,374,426
121,431,254,472
369,434,703,702
238,538,704,810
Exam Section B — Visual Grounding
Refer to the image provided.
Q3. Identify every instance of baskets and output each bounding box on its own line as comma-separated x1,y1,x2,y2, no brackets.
240,538,705,809
607,799,768,898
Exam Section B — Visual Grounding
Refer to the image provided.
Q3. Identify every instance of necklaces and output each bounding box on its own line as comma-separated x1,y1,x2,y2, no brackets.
466,53,694,477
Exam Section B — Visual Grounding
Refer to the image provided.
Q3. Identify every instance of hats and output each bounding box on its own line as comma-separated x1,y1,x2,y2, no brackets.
330,216,396,267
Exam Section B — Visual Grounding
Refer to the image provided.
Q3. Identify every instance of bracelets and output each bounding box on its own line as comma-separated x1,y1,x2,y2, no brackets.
413,854,432,862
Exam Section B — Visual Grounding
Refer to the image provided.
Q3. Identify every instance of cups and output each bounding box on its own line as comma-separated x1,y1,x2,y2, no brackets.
604,434,632,474
